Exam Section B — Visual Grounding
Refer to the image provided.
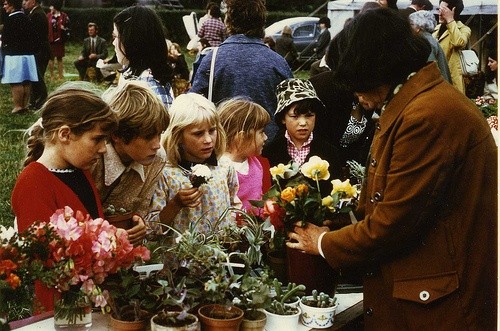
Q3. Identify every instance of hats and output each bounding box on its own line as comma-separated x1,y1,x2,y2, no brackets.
274,78,328,117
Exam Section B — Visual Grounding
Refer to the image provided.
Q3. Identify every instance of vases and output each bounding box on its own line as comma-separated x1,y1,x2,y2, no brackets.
54,288,93,328
288,238,339,298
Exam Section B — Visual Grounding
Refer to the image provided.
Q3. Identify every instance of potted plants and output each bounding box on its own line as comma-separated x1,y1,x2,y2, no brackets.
109,206,339,331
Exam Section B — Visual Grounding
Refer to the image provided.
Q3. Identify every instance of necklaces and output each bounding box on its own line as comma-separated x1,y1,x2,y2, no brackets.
351,96,359,110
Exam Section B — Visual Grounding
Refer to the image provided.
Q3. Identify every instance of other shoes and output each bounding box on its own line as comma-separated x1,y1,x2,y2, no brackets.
28,95,46,109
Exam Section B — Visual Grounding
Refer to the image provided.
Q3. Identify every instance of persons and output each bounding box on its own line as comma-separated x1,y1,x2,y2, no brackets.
218,96,271,221
88,82,170,248
286,8,498,331
309,70,377,186
11,89,119,316
264,17,353,76
266,78,337,198
197,2,229,48
96,38,190,87
142,93,246,269
102,5,175,111
188,0,294,143
360,0,499,104
0,0,70,113
74,23,108,82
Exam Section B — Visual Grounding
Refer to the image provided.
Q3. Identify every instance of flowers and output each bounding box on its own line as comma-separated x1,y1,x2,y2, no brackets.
0,207,151,323
263,156,364,228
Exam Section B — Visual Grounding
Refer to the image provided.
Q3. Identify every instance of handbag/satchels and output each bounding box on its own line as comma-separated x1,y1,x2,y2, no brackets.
459,26,480,76
61,13,73,43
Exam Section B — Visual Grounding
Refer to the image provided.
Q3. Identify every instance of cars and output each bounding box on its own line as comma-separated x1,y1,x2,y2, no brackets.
262,15,323,56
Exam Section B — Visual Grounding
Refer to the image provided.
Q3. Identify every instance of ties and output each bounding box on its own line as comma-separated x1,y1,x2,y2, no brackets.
92,38,95,53
